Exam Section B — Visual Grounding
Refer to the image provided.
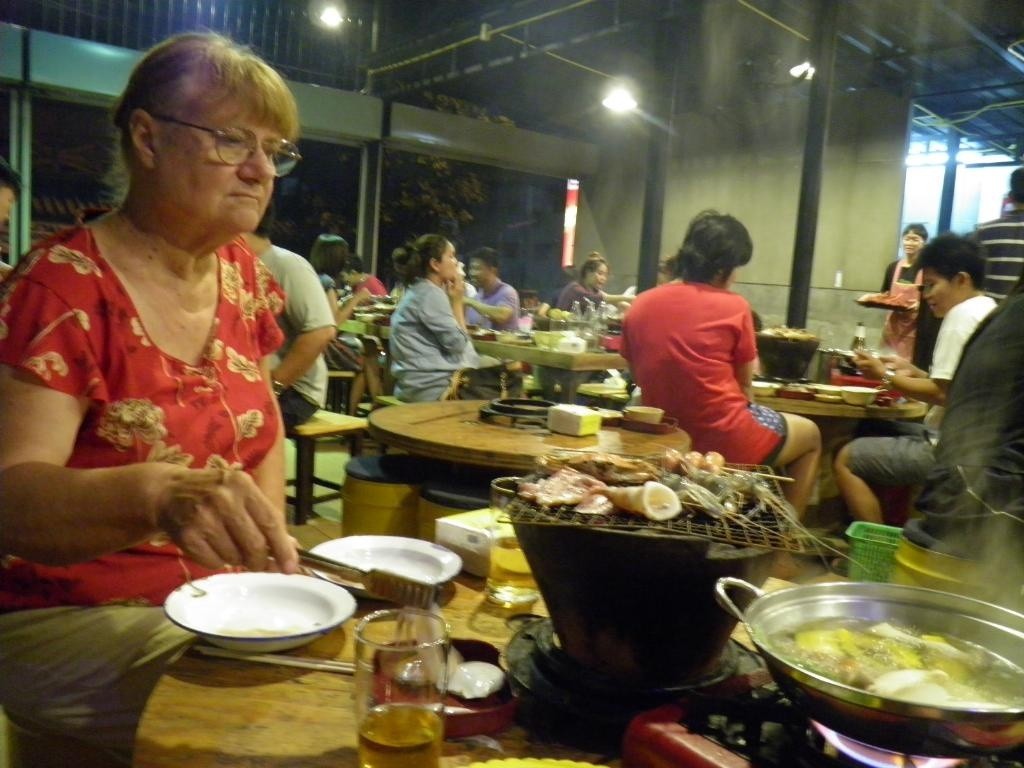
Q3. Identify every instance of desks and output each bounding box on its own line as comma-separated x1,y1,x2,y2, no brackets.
366,399,688,475
751,393,928,504
133,542,853,768
337,315,629,404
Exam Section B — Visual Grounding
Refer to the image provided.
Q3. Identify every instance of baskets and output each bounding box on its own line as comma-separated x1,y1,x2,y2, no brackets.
846,521,906,582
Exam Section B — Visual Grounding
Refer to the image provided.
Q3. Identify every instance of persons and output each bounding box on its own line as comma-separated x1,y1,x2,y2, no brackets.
0,33,303,745
833,232,1000,526
973,168,1024,304
339,249,389,299
392,242,520,330
0,157,23,280
878,223,928,356
390,235,529,398
309,234,384,419
908,271,1024,578
537,250,666,322
254,244,338,434
621,209,821,525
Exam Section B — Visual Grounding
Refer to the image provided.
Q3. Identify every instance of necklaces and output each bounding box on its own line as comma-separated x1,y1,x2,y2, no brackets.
359,273,367,282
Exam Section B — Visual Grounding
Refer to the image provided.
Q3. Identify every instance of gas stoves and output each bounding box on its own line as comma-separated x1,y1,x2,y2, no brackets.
623,660,1024,768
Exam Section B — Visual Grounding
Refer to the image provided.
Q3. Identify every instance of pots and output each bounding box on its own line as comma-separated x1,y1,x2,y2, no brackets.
713,577,1024,757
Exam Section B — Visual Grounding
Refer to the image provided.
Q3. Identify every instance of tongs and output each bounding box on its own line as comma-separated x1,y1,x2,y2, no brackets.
264,544,435,609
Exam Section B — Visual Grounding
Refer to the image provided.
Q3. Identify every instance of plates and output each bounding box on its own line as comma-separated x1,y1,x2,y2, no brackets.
353,295,395,322
307,536,462,598
164,571,357,652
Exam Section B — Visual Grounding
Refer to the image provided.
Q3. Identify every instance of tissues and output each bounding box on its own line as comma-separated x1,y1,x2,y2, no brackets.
558,330,588,352
548,402,601,437
603,368,627,389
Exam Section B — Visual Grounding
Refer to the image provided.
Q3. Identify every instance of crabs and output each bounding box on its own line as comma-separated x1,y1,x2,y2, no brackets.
536,447,665,486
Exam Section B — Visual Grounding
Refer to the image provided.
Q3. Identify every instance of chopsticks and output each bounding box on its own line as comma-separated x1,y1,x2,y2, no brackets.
193,645,355,674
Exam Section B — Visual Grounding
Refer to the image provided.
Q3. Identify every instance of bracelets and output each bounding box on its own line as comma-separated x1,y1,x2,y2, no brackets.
272,379,284,396
881,371,894,392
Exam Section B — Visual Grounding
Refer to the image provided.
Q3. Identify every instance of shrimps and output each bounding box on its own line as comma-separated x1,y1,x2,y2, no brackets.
645,439,803,529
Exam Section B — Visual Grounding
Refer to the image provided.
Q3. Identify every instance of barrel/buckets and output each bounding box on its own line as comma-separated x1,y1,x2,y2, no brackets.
755,333,820,382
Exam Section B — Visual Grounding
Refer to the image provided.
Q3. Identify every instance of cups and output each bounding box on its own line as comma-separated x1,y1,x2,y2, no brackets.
623,406,665,424
352,607,449,768
485,476,542,609
515,307,535,332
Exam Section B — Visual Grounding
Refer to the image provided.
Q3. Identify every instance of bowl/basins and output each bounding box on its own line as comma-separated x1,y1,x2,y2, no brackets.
493,331,528,342
752,381,782,396
839,386,879,404
534,331,572,349
817,385,841,395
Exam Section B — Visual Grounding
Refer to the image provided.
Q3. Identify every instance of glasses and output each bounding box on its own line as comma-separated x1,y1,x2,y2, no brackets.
148,113,302,177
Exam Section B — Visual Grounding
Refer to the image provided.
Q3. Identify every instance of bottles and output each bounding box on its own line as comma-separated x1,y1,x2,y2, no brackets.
850,321,866,351
569,300,609,352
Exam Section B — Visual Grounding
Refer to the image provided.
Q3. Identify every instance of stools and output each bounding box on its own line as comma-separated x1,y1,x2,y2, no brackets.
283,409,367,528
340,454,489,541
